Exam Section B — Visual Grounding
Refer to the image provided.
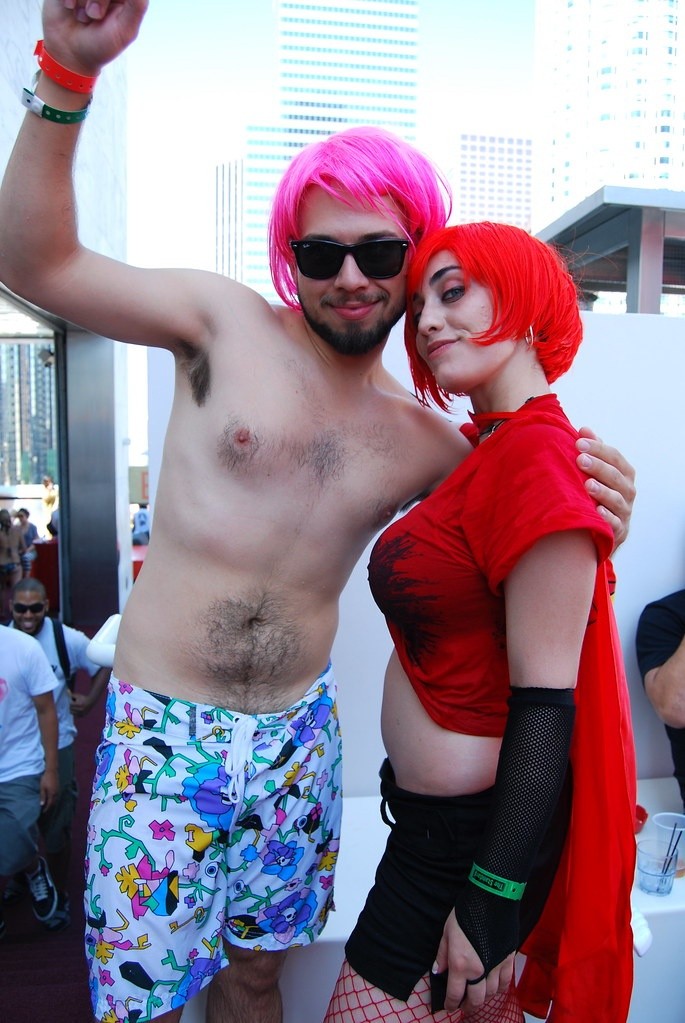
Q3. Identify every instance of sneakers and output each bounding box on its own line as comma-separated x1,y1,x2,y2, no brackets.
23,856,58,921
0,920,8,940
1,872,28,904
45,888,72,934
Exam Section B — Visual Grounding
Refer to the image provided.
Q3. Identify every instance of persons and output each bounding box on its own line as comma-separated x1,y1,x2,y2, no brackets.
0,508,38,620
132,501,149,544
47,510,58,538
44,477,55,510
325,220,635,1023
0,0,637,1023
0,579,112,940
635,587,685,814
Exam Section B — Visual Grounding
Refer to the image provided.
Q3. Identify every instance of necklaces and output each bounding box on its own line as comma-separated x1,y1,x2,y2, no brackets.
478,396,535,437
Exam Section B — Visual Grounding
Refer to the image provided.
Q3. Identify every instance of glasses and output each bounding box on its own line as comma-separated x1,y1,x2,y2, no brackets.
17,514,23,517
12,602,46,614
290,238,410,280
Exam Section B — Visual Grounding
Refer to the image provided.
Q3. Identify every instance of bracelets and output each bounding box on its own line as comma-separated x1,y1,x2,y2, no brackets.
468,861,526,902
20,69,93,124
34,40,101,92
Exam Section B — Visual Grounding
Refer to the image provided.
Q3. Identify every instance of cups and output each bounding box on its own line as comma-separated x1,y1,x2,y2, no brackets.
636,840,679,897
652,812,685,871
